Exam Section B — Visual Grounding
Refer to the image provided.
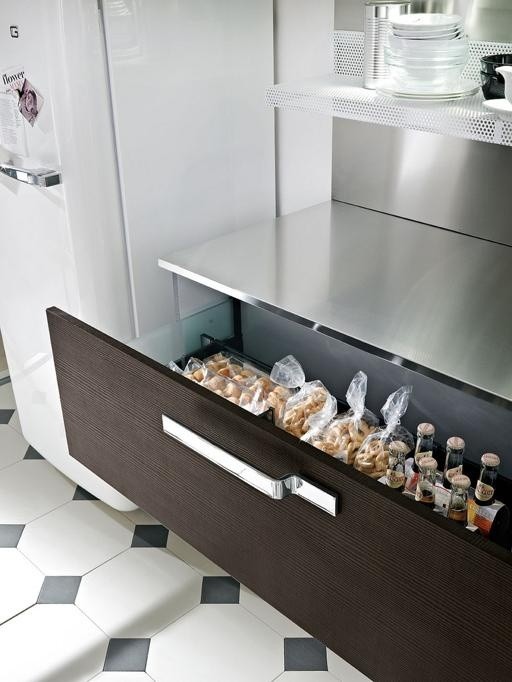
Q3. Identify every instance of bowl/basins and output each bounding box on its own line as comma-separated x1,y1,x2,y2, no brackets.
480,54,512,100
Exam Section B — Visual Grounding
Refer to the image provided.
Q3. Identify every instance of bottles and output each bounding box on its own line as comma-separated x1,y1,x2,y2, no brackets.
379,419,502,536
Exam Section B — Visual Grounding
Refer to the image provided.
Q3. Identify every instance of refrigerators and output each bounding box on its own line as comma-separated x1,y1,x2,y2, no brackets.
1,1,278,516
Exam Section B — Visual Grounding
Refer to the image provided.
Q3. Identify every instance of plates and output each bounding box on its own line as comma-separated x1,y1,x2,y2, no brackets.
483,98,512,123
375,13,480,113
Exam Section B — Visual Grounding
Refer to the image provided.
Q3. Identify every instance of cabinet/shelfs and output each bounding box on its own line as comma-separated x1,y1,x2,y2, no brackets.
46,75,512,681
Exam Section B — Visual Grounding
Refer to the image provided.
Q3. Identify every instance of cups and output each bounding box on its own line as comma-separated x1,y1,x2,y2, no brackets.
495,66,512,105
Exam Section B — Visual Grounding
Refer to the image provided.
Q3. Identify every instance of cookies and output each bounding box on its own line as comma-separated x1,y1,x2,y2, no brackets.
185,356,291,409
317,412,376,466
353,429,410,480
283,386,328,438
